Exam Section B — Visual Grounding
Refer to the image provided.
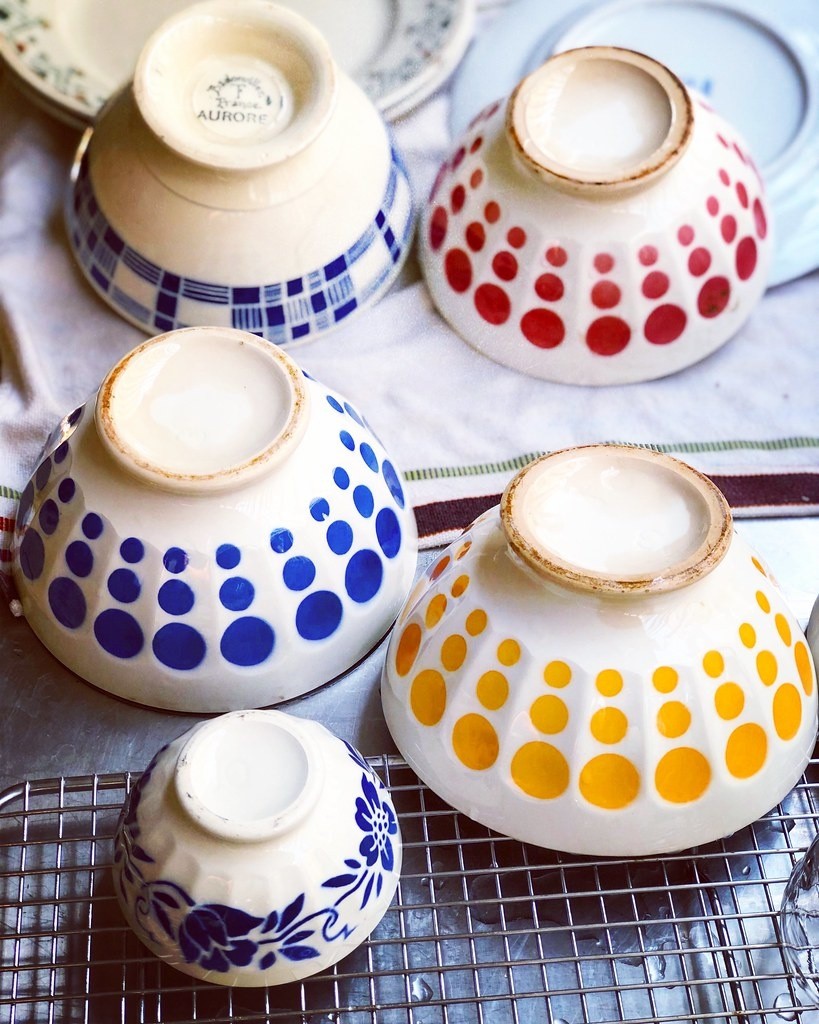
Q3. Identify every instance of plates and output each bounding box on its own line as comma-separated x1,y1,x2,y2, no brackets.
0,0,477,125
448,0,819,291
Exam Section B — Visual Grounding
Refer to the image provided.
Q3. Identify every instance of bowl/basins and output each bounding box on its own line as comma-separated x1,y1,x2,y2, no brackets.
10,326,419,713
65,0,417,348
111,709,403,987
379,445,819,857
417,46,771,386
